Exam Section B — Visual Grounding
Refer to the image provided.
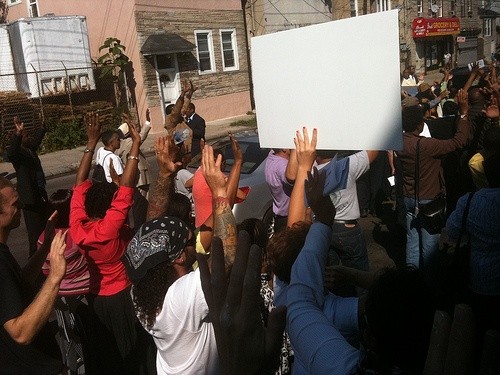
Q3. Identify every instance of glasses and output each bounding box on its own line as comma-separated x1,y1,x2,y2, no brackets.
185,233,196,248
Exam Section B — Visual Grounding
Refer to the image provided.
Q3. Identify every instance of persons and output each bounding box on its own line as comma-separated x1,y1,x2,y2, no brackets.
0,60,500,375
4,114,54,261
122,135,239,374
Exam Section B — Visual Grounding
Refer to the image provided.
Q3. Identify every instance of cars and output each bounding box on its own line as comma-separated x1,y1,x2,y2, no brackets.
171,128,280,252
437,66,471,99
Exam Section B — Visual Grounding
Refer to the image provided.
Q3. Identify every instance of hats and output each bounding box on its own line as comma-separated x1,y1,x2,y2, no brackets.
119,122,129,137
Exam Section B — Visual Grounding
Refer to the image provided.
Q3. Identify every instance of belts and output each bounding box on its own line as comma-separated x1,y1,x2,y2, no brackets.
335,220,357,223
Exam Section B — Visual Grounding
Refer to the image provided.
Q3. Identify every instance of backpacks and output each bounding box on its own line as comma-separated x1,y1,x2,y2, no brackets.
90,149,112,184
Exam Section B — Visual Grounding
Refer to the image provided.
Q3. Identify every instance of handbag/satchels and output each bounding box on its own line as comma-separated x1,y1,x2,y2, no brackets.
416,191,448,232
